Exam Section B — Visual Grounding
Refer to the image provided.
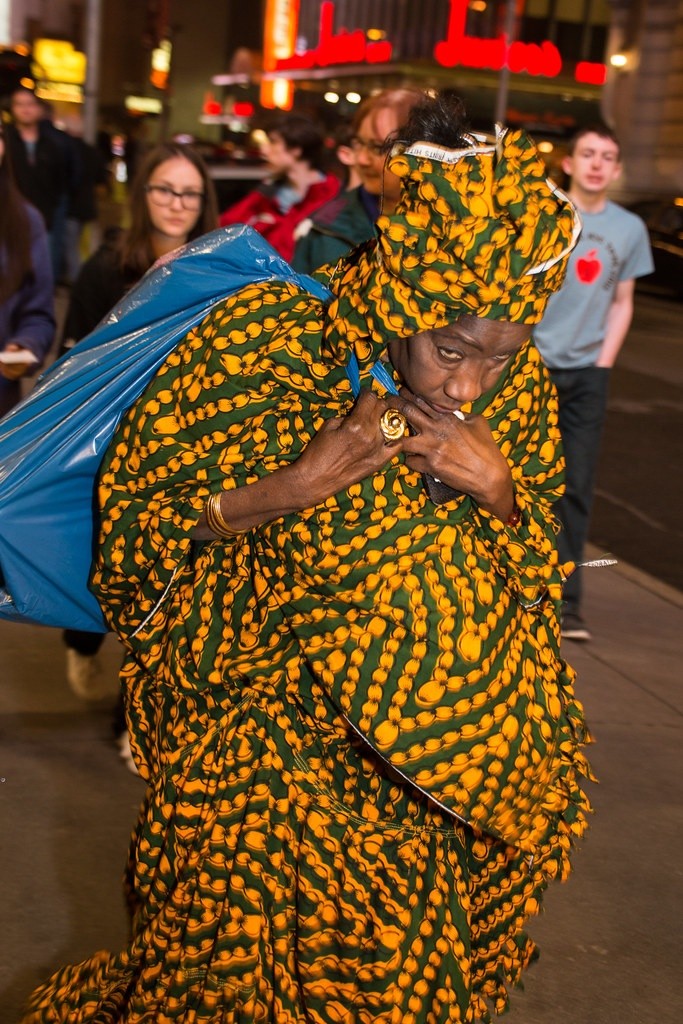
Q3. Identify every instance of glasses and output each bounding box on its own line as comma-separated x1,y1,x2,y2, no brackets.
349,137,391,157
143,181,205,211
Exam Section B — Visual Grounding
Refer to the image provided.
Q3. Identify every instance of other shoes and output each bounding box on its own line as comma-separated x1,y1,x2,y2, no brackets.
556,614,594,644
65,648,105,701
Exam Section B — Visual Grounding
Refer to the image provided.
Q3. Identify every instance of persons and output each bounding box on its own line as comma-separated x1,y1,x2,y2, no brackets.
528,117,654,642
21,116,598,1024
0,46,457,779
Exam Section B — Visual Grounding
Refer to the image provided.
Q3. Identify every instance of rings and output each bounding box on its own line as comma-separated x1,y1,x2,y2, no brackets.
380,410,406,441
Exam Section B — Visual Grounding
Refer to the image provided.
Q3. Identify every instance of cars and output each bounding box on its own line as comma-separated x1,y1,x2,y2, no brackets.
622,195,683,299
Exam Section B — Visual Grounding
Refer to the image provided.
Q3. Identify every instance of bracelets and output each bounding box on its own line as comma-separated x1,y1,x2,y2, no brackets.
207,492,250,538
